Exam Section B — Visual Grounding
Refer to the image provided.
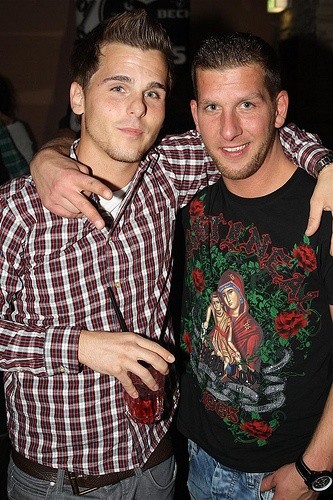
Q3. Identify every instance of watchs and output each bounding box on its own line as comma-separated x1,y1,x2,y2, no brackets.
295,454,333,491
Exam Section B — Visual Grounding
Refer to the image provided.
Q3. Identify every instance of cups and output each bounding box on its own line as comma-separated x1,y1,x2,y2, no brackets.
124,365,165,423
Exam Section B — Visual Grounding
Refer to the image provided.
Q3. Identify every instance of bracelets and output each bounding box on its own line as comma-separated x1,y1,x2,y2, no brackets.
315,154,332,174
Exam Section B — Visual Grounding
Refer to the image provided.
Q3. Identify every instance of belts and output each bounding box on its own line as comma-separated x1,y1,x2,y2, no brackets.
11,432,173,488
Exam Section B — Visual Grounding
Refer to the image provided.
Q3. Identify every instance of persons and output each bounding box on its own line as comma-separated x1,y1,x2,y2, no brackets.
1,75,33,180
30,34,333,500
0,8,332,499
